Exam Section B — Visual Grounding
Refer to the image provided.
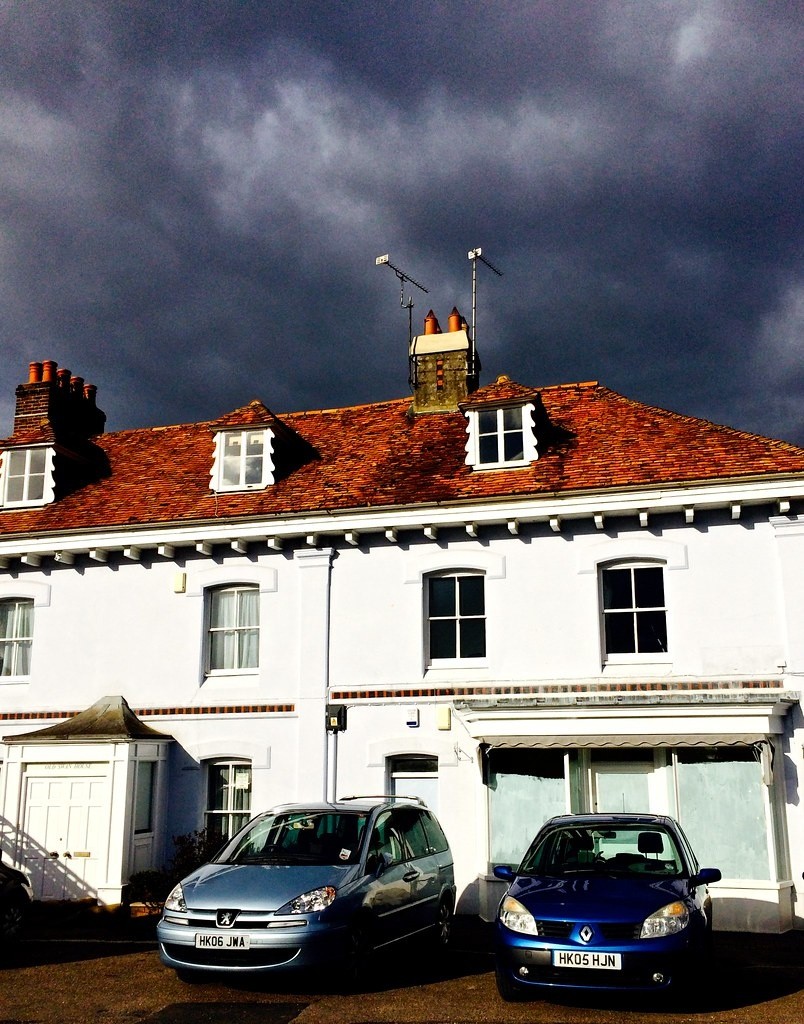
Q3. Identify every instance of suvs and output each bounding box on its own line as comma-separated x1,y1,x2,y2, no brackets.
155,794,459,986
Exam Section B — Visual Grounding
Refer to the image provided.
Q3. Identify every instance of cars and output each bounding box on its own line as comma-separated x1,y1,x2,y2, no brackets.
492,812,722,1000
0,849,33,958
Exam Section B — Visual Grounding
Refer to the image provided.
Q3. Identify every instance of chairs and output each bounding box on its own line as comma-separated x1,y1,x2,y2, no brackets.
291,828,358,855
356,825,387,860
568,836,594,868
638,832,664,862
